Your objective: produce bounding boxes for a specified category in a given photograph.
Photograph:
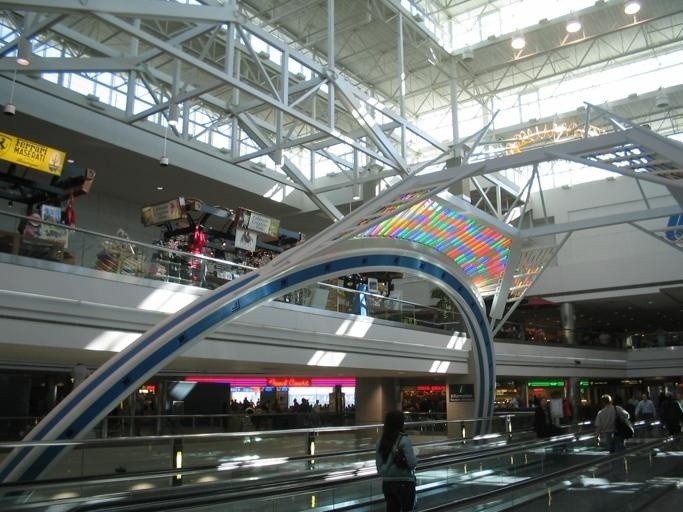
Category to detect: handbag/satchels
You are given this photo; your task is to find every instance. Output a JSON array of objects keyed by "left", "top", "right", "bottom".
[
  {"left": 617, "top": 416, "right": 634, "bottom": 439},
  {"left": 393, "top": 446, "right": 407, "bottom": 468}
]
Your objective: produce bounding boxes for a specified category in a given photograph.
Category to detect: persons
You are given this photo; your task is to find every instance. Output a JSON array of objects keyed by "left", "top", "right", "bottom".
[
  {"left": 230, "top": 397, "right": 355, "bottom": 412},
  {"left": 535, "top": 398, "right": 548, "bottom": 437},
  {"left": 376, "top": 411, "right": 416, "bottom": 512},
  {"left": 352, "top": 283, "right": 367, "bottom": 315},
  {"left": 657, "top": 392, "right": 683, "bottom": 434},
  {"left": 593, "top": 394, "right": 630, "bottom": 448},
  {"left": 635, "top": 393, "right": 656, "bottom": 418}
]
